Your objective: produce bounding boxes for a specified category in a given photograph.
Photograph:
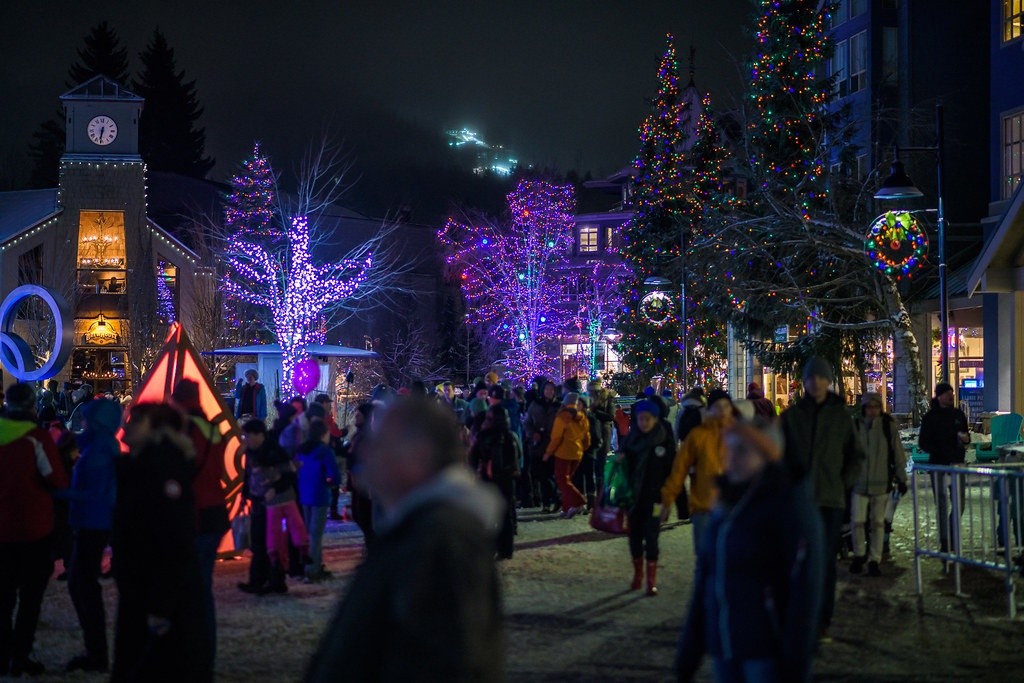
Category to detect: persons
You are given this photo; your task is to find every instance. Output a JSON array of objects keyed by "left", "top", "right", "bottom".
[
  {"left": 234, "top": 369, "right": 267, "bottom": 427},
  {"left": 918, "top": 383, "right": 971, "bottom": 566},
  {"left": 287, "top": 390, "right": 508, "bottom": 683},
  {"left": 240, "top": 380, "right": 428, "bottom": 602},
  {"left": 674, "top": 418, "right": 823, "bottom": 683},
  {"left": 0, "top": 377, "right": 229, "bottom": 683},
  {"left": 109, "top": 277, "right": 122, "bottom": 292},
  {"left": 431, "top": 370, "right": 616, "bottom": 559},
  {"left": 617, "top": 359, "right": 908, "bottom": 595}
]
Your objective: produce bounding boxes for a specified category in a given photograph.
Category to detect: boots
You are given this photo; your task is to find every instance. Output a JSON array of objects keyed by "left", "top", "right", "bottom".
[
  {"left": 630, "top": 557, "right": 644, "bottom": 588},
  {"left": 647, "top": 559, "right": 659, "bottom": 597}
]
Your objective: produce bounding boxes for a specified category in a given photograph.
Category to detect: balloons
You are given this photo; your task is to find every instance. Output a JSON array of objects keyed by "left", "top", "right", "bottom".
[
  {"left": 291, "top": 359, "right": 321, "bottom": 397},
  {"left": 864, "top": 210, "right": 929, "bottom": 282},
  {"left": 637, "top": 292, "right": 677, "bottom": 330}
]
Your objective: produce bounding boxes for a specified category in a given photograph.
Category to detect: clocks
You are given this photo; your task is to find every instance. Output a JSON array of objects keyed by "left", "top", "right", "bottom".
[{"left": 86, "top": 115, "right": 119, "bottom": 147}]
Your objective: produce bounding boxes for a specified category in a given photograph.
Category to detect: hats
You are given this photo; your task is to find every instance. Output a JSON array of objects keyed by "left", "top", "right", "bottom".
[
  {"left": 801, "top": 356, "right": 833, "bottom": 384},
  {"left": 587, "top": 378, "right": 603, "bottom": 392},
  {"left": 635, "top": 400, "right": 659, "bottom": 417},
  {"left": 563, "top": 393, "right": 578, "bottom": 406},
  {"left": 935, "top": 383, "right": 952, "bottom": 396},
  {"left": 728, "top": 416, "right": 785, "bottom": 464},
  {"left": 485, "top": 371, "right": 498, "bottom": 384},
  {"left": 488, "top": 387, "right": 503, "bottom": 399}
]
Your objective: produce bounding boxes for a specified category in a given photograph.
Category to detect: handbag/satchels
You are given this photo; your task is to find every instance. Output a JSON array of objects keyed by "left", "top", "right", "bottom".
[{"left": 588, "top": 481, "right": 631, "bottom": 534}]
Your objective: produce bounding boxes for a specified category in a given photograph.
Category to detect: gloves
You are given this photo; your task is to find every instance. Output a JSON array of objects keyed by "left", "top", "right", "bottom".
[{"left": 897, "top": 481, "right": 908, "bottom": 496}]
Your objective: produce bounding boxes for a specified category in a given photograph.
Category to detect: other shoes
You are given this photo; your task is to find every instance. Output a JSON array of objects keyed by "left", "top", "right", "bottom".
[
  {"left": 9, "top": 657, "right": 46, "bottom": 677},
  {"left": 541, "top": 506, "right": 552, "bottom": 514},
  {"left": 67, "top": 656, "right": 108, "bottom": 672},
  {"left": 496, "top": 553, "right": 512, "bottom": 561},
  {"left": 850, "top": 559, "right": 879, "bottom": 576},
  {"left": 237, "top": 580, "right": 288, "bottom": 596},
  {"left": 881, "top": 553, "right": 896, "bottom": 565},
  {"left": 560, "top": 501, "right": 587, "bottom": 518}
]
